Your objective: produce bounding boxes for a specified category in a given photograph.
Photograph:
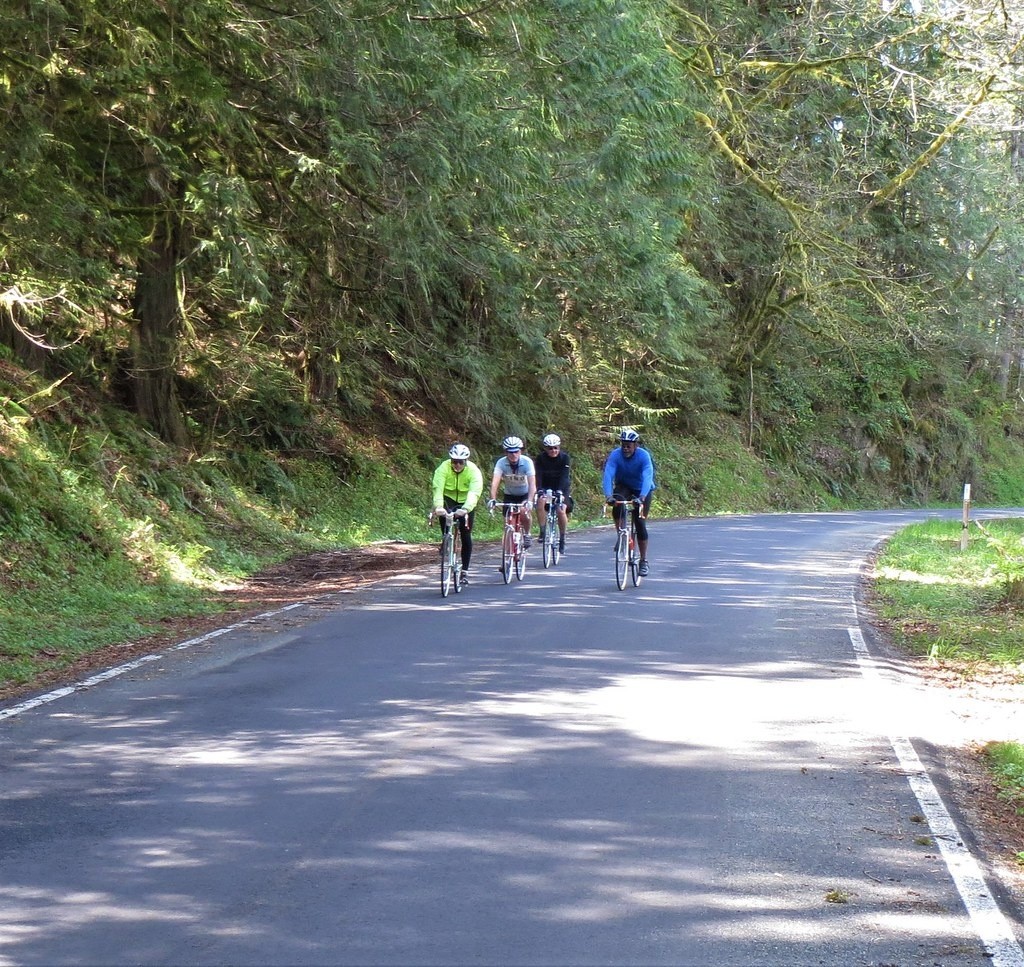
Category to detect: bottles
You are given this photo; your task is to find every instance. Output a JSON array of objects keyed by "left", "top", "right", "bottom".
[{"left": 629, "top": 538, "right": 634, "bottom": 551}]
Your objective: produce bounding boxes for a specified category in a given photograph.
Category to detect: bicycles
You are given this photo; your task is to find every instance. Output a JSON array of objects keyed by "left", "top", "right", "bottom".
[
  {"left": 601, "top": 499, "right": 647, "bottom": 592},
  {"left": 533, "top": 489, "right": 566, "bottom": 567},
  {"left": 486, "top": 499, "right": 534, "bottom": 584},
  {"left": 428, "top": 508, "right": 471, "bottom": 596}
]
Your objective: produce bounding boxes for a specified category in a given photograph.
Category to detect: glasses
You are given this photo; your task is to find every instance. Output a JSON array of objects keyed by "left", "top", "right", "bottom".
[
  {"left": 546, "top": 446, "right": 557, "bottom": 450},
  {"left": 506, "top": 451, "right": 518, "bottom": 453},
  {"left": 453, "top": 460, "right": 464, "bottom": 464},
  {"left": 622, "top": 444, "right": 635, "bottom": 448}
]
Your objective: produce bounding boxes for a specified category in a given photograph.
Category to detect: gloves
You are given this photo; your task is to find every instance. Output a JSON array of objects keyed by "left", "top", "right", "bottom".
[
  {"left": 635, "top": 496, "right": 646, "bottom": 504},
  {"left": 606, "top": 497, "right": 616, "bottom": 506}
]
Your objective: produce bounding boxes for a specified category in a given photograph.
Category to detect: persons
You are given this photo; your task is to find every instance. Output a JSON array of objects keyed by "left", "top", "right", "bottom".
[
  {"left": 432, "top": 444, "right": 483, "bottom": 584},
  {"left": 534, "top": 434, "right": 570, "bottom": 554},
  {"left": 602, "top": 429, "right": 657, "bottom": 576},
  {"left": 490, "top": 436, "right": 536, "bottom": 573}
]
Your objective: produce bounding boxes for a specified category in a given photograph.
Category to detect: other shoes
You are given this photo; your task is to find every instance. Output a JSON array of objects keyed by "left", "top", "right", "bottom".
[
  {"left": 639, "top": 559, "right": 650, "bottom": 576},
  {"left": 524, "top": 534, "right": 532, "bottom": 548},
  {"left": 538, "top": 531, "right": 546, "bottom": 542},
  {"left": 559, "top": 535, "right": 566, "bottom": 554},
  {"left": 440, "top": 541, "right": 448, "bottom": 556},
  {"left": 499, "top": 563, "right": 508, "bottom": 573},
  {"left": 614, "top": 535, "right": 626, "bottom": 551},
  {"left": 460, "top": 571, "right": 468, "bottom": 584}
]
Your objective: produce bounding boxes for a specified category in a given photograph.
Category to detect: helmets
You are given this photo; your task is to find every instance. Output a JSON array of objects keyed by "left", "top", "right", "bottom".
[
  {"left": 621, "top": 430, "right": 639, "bottom": 441},
  {"left": 449, "top": 445, "right": 470, "bottom": 460},
  {"left": 503, "top": 437, "right": 523, "bottom": 451},
  {"left": 543, "top": 434, "right": 561, "bottom": 446}
]
